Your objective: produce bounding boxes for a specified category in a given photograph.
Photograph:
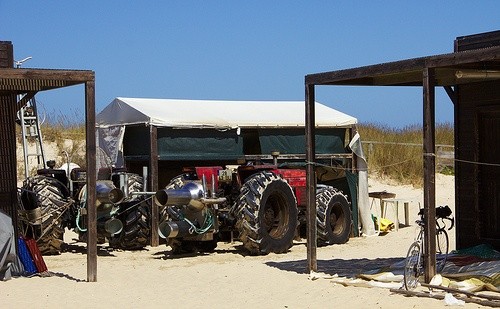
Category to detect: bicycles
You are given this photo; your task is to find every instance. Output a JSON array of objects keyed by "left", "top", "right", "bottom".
[{"left": 399, "top": 205, "right": 455, "bottom": 292}]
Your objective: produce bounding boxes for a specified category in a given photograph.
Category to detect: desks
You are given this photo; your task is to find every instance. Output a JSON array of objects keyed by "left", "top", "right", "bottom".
[
  {"left": 368, "top": 191, "right": 397, "bottom": 221},
  {"left": 382, "top": 198, "right": 421, "bottom": 231}
]
